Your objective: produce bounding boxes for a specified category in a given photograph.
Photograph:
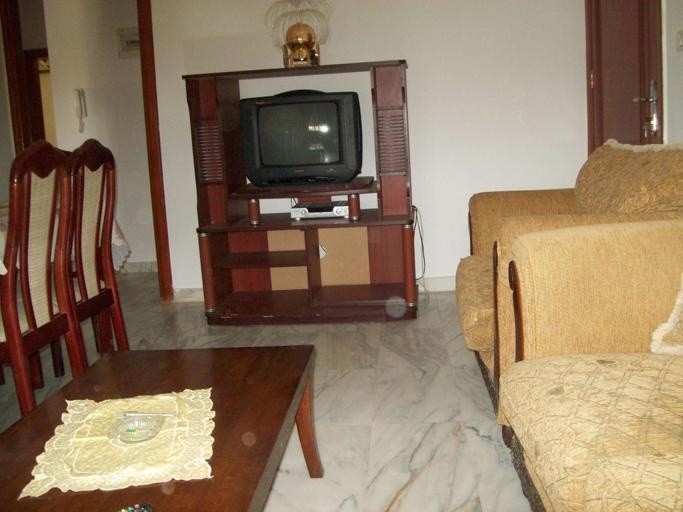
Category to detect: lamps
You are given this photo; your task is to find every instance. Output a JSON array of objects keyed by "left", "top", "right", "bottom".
[{"left": 261, "top": 0, "right": 334, "bottom": 70}]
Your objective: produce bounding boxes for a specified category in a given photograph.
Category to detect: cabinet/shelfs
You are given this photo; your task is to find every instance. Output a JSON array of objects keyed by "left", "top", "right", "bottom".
[{"left": 172, "top": 58, "right": 419, "bottom": 329}]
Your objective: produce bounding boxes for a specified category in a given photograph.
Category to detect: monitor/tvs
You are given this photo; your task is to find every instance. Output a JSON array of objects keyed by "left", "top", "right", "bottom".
[{"left": 238, "top": 89, "right": 363, "bottom": 188}]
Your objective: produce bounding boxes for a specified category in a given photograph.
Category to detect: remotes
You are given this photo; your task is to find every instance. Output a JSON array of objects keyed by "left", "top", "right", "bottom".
[{"left": 119, "top": 502, "right": 155, "bottom": 512}]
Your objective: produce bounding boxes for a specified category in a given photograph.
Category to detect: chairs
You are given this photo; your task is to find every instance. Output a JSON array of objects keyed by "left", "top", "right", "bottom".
[{"left": 1, "top": 137, "right": 131, "bottom": 420}]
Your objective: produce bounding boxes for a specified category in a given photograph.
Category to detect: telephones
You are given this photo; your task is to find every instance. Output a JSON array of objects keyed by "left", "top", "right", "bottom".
[{"left": 72, "top": 88, "right": 87, "bottom": 119}]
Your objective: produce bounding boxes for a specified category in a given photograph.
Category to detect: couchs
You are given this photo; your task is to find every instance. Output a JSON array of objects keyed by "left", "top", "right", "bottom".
[
  {"left": 499, "top": 217, "right": 683, "bottom": 512},
  {"left": 453, "top": 140, "right": 683, "bottom": 422}
]
